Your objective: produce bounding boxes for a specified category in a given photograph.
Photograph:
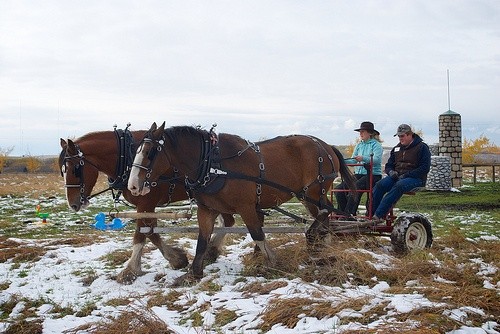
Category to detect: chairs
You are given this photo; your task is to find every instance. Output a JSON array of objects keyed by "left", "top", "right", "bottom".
[{"left": 384, "top": 185, "right": 423, "bottom": 220}]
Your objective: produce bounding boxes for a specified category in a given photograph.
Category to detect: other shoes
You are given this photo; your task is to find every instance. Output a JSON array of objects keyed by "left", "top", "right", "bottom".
[
  {"left": 338, "top": 215, "right": 347, "bottom": 221},
  {"left": 348, "top": 215, "right": 354, "bottom": 219},
  {"left": 372, "top": 215, "right": 384, "bottom": 225}
]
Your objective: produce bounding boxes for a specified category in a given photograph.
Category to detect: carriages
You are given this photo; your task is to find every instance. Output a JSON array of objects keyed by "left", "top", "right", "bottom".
[{"left": 58, "top": 122, "right": 434, "bottom": 284}]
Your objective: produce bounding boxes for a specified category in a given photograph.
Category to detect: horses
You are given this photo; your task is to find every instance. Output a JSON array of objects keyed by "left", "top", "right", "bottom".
[
  {"left": 58, "top": 129, "right": 265, "bottom": 285},
  {"left": 127, "top": 121, "right": 359, "bottom": 279}
]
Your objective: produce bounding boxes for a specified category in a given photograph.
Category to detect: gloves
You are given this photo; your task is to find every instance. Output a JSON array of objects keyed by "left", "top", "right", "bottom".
[
  {"left": 398, "top": 174, "right": 407, "bottom": 180},
  {"left": 388, "top": 170, "right": 399, "bottom": 180}
]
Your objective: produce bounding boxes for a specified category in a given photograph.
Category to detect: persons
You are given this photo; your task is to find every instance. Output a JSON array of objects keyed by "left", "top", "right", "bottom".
[
  {"left": 334, "top": 121, "right": 384, "bottom": 220},
  {"left": 365, "top": 124, "right": 431, "bottom": 222}
]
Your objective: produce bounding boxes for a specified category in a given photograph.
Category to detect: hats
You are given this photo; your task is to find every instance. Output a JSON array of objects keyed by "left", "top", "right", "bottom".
[
  {"left": 394, "top": 124, "right": 411, "bottom": 137},
  {"left": 354, "top": 122, "right": 380, "bottom": 135}
]
